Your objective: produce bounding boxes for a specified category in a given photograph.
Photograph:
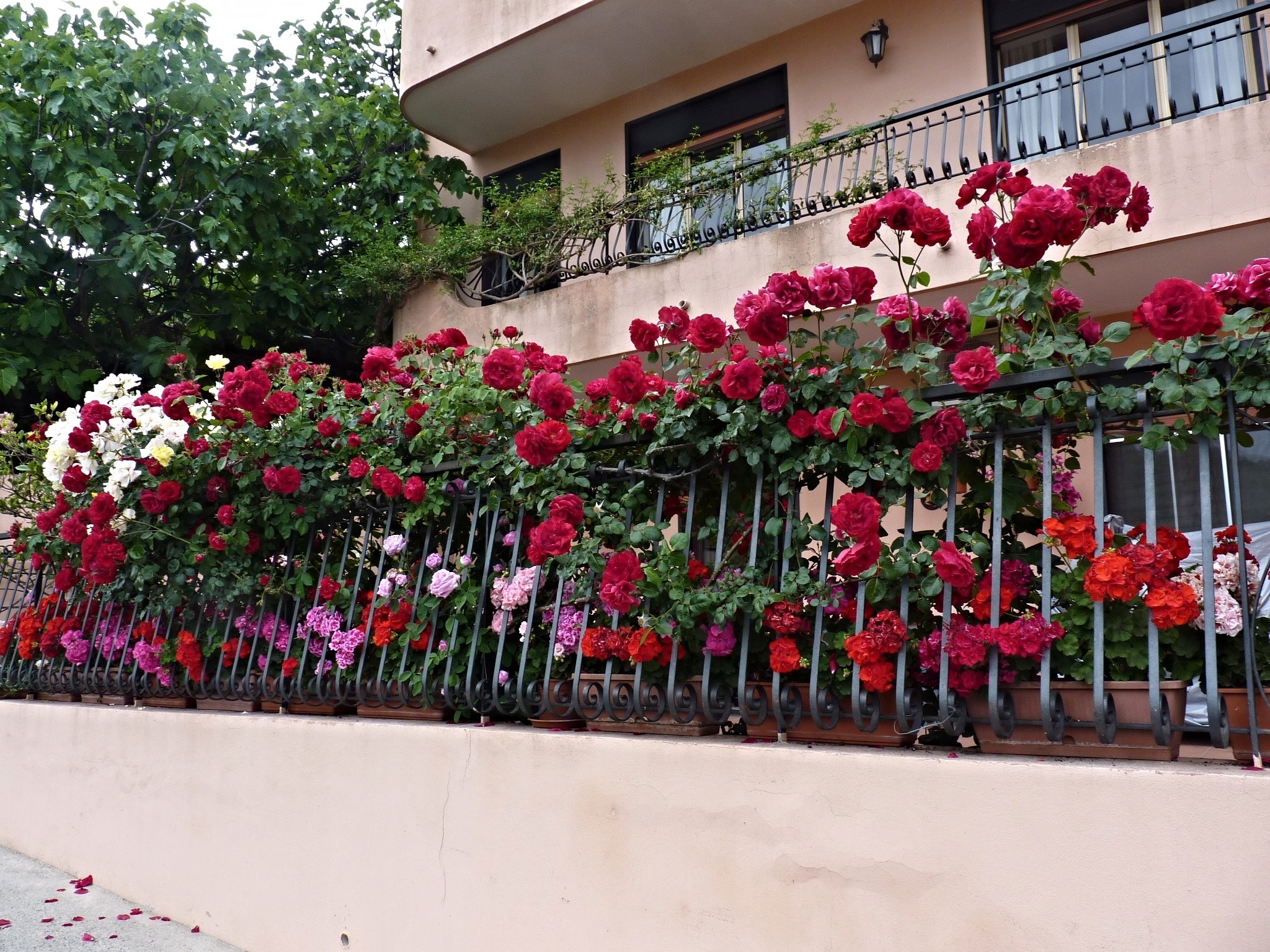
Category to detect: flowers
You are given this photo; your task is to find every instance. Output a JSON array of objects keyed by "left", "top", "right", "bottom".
[{"left": 0, "top": 161, "right": 1270, "bottom": 722}]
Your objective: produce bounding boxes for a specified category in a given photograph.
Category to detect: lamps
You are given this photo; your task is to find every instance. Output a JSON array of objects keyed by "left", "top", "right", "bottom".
[{"left": 860, "top": 19, "right": 889, "bottom": 69}]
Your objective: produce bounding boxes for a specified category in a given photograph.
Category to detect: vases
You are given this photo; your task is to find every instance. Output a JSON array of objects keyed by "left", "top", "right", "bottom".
[
  {"left": 527, "top": 679, "right": 586, "bottom": 730},
  {"left": 1217, "top": 689, "right": 1270, "bottom": 765},
  {"left": 34, "top": 666, "right": 77, "bottom": 702},
  {"left": 354, "top": 674, "right": 462, "bottom": 722},
  {"left": 193, "top": 669, "right": 258, "bottom": 712},
  {"left": 966, "top": 681, "right": 1187, "bottom": 761},
  {"left": 572, "top": 673, "right": 721, "bottom": 736},
  {"left": 76, "top": 667, "right": 134, "bottom": 705},
  {"left": 745, "top": 682, "right": 915, "bottom": 746},
  {"left": 255, "top": 672, "right": 337, "bottom": 716},
  {"left": 134, "top": 673, "right": 191, "bottom": 708}
]
